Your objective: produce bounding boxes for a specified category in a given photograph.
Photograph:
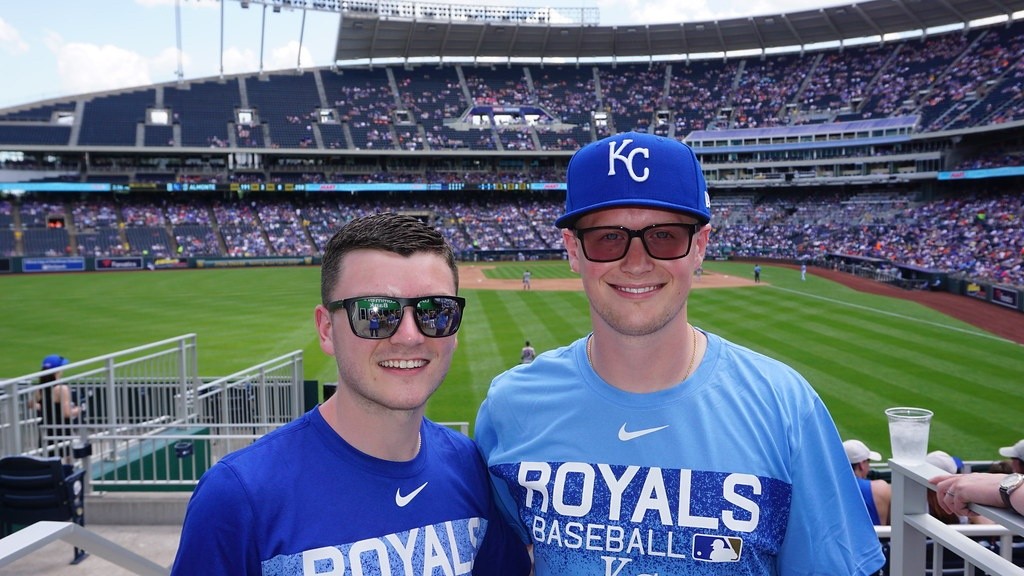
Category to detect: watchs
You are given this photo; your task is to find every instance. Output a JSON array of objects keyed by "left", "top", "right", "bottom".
[{"left": 999, "top": 472, "right": 1024, "bottom": 513}]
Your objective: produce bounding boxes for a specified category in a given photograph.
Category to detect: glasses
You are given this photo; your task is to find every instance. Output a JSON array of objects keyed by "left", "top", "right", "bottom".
[
  {"left": 574, "top": 224, "right": 702, "bottom": 262},
  {"left": 325, "top": 295, "right": 466, "bottom": 339}
]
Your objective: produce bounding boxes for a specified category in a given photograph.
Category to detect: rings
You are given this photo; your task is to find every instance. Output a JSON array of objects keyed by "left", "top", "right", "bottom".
[{"left": 947, "top": 490, "right": 954, "bottom": 496}]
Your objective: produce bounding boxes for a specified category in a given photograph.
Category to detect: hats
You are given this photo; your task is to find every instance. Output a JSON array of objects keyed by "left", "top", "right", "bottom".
[
  {"left": 999, "top": 439, "right": 1024, "bottom": 460},
  {"left": 555, "top": 133, "right": 709, "bottom": 226},
  {"left": 843, "top": 439, "right": 881, "bottom": 463},
  {"left": 43, "top": 355, "right": 68, "bottom": 367},
  {"left": 927, "top": 451, "right": 964, "bottom": 474}
]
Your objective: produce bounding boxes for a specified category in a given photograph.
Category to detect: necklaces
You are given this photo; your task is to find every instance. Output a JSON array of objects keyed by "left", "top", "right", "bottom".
[{"left": 588, "top": 323, "right": 697, "bottom": 383}]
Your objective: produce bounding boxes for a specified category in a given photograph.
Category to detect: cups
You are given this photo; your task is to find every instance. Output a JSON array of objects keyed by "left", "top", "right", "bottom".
[{"left": 885, "top": 407, "right": 933, "bottom": 466}]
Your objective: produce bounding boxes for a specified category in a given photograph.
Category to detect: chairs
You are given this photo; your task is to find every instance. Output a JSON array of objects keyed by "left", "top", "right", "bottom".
[{"left": 0, "top": 456, "right": 90, "bottom": 565}]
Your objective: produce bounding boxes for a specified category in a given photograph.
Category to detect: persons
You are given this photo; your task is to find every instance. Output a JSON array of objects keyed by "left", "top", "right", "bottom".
[
  {"left": 754, "top": 264, "right": 761, "bottom": 282},
  {"left": 168, "top": 29, "right": 1024, "bottom": 151},
  {"left": 842, "top": 439, "right": 891, "bottom": 525},
  {"left": 697, "top": 267, "right": 703, "bottom": 280},
  {"left": 0, "top": 155, "right": 1024, "bottom": 289},
  {"left": 929, "top": 472, "right": 1024, "bottom": 517},
  {"left": 170, "top": 210, "right": 532, "bottom": 576},
  {"left": 473, "top": 129, "right": 886, "bottom": 576},
  {"left": 27, "top": 354, "right": 82, "bottom": 457},
  {"left": 926, "top": 450, "right": 964, "bottom": 570},
  {"left": 998, "top": 440, "right": 1024, "bottom": 474},
  {"left": 422, "top": 309, "right": 459, "bottom": 335},
  {"left": 369, "top": 308, "right": 398, "bottom": 337},
  {"left": 801, "top": 262, "right": 806, "bottom": 280},
  {"left": 521, "top": 341, "right": 535, "bottom": 363},
  {"left": 523, "top": 269, "right": 530, "bottom": 290}
]
